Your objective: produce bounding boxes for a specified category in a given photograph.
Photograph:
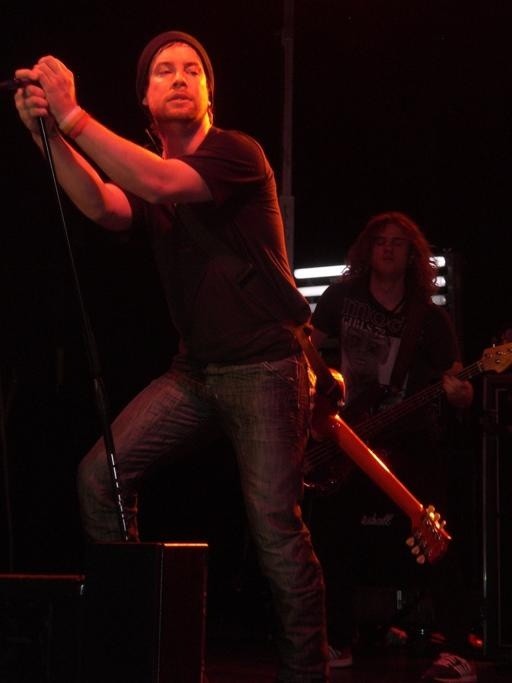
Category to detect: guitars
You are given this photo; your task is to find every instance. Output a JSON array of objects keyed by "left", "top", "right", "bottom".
[
  {"left": 306, "top": 333, "right": 453, "bottom": 565},
  {"left": 302, "top": 339, "right": 512, "bottom": 506}
]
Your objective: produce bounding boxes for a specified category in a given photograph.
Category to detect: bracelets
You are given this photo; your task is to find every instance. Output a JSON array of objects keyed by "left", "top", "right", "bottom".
[{"left": 60, "top": 105, "right": 92, "bottom": 141}]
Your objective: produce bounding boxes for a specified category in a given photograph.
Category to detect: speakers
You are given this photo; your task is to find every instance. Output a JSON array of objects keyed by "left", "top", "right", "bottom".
[
  {"left": 0, "top": 573, "right": 86, "bottom": 682},
  {"left": 85, "top": 539, "right": 210, "bottom": 682}
]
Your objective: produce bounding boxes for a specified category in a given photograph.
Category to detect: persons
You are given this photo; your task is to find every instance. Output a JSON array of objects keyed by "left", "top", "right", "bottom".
[
  {"left": 302, "top": 212, "right": 488, "bottom": 682},
  {"left": 11, "top": 30, "right": 330, "bottom": 680}
]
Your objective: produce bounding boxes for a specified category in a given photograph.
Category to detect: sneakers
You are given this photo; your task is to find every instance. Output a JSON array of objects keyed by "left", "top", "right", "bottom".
[
  {"left": 327, "top": 645, "right": 354, "bottom": 668},
  {"left": 422, "top": 652, "right": 478, "bottom": 683}
]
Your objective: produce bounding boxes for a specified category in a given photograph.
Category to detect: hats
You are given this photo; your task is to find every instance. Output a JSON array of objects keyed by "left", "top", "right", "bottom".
[{"left": 135, "top": 30, "right": 216, "bottom": 107}]
